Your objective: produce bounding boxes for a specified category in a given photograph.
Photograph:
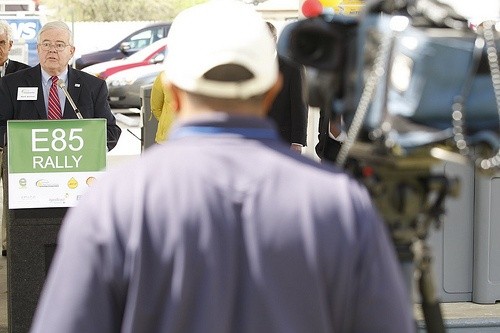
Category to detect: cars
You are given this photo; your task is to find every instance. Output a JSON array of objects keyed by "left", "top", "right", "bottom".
[
  {"left": 70, "top": 23, "right": 172, "bottom": 75},
  {"left": 77, "top": 35, "right": 166, "bottom": 111}
]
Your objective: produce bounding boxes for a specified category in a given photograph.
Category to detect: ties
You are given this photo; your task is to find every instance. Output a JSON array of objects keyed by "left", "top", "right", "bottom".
[{"left": 47, "top": 75, "right": 63, "bottom": 121}]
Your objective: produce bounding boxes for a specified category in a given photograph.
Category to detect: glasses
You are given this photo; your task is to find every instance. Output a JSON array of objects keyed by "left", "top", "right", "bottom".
[{"left": 38, "top": 42, "right": 72, "bottom": 51}]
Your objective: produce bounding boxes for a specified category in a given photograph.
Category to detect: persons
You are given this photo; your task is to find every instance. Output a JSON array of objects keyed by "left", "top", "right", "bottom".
[
  {"left": 148, "top": 71, "right": 173, "bottom": 144},
  {"left": 31, "top": 0, "right": 418, "bottom": 333},
  {"left": 315, "top": 107, "right": 355, "bottom": 166},
  {"left": 0, "top": 21, "right": 122, "bottom": 152},
  {"left": 266, "top": 22, "right": 308, "bottom": 156},
  {"left": 0, "top": 19, "right": 31, "bottom": 256}
]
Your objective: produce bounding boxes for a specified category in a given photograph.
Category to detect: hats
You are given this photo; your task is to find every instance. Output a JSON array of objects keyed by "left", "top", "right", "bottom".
[{"left": 164, "top": 0, "right": 279, "bottom": 100}]
